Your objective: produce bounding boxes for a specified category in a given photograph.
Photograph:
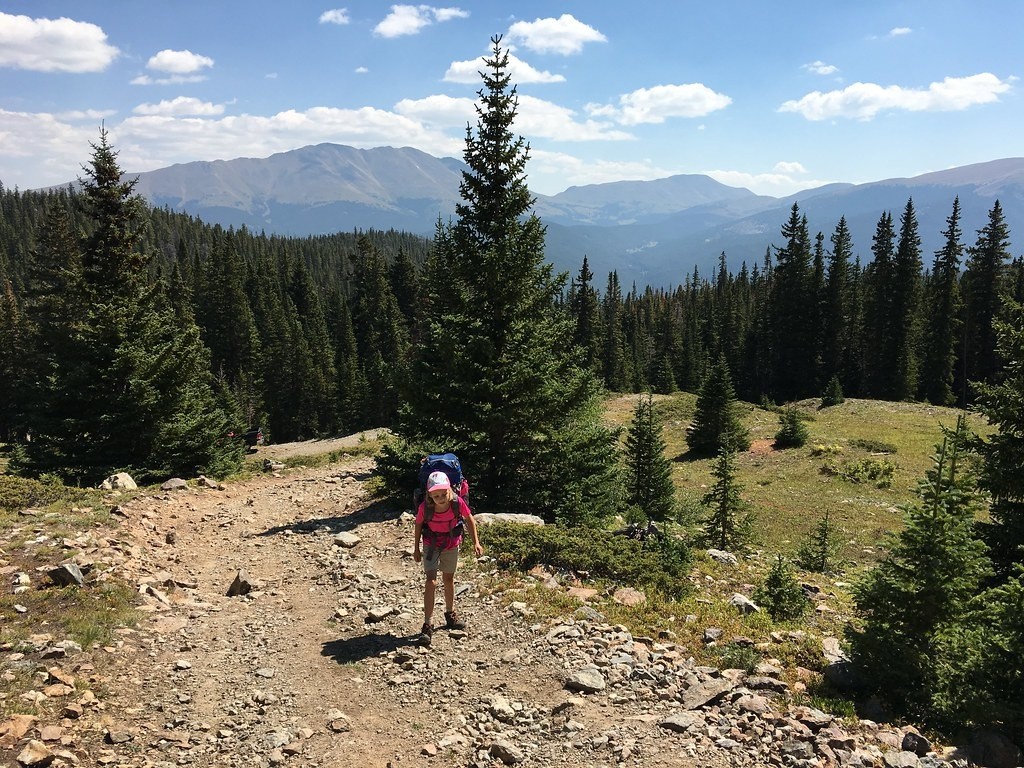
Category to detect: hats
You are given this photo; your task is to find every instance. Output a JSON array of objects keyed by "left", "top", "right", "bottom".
[{"left": 427, "top": 471, "right": 450, "bottom": 492}]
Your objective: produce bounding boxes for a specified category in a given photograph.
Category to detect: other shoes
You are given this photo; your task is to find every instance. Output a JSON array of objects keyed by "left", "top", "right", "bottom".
[
  {"left": 419, "top": 623, "right": 432, "bottom": 644},
  {"left": 444, "top": 613, "right": 465, "bottom": 629}
]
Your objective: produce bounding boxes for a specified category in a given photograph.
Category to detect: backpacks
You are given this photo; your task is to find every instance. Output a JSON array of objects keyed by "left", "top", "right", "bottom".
[{"left": 419, "top": 453, "right": 469, "bottom": 537}]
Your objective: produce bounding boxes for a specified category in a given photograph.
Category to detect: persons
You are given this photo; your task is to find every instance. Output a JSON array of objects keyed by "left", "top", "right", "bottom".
[{"left": 413, "top": 472, "right": 483, "bottom": 644}]
